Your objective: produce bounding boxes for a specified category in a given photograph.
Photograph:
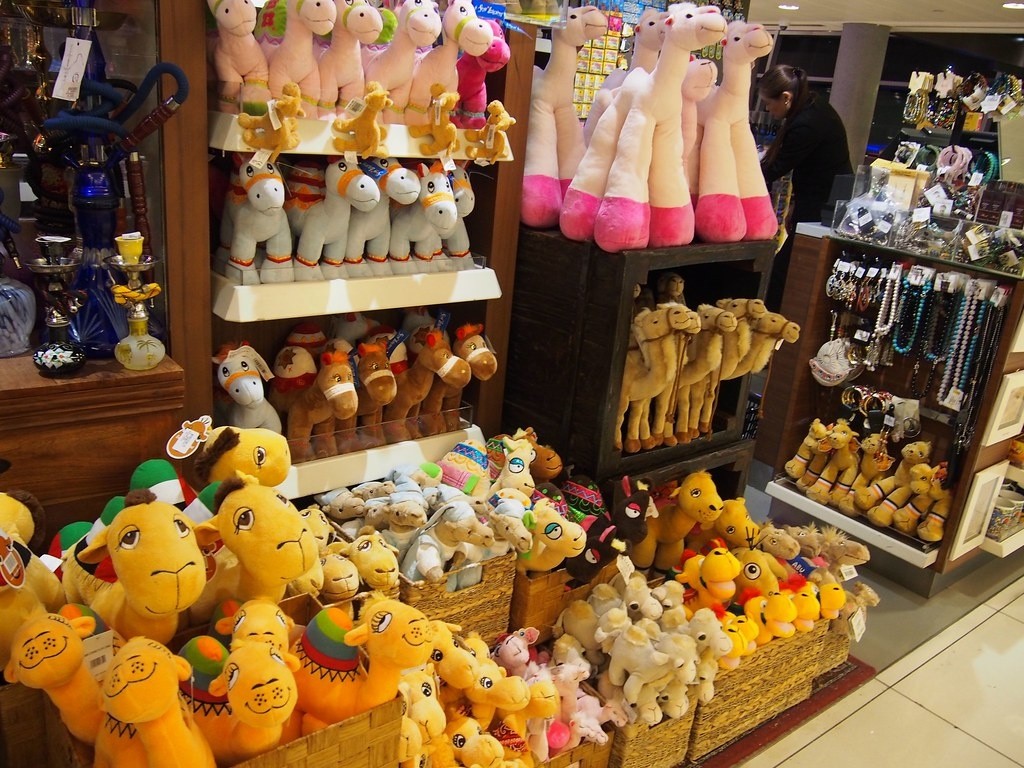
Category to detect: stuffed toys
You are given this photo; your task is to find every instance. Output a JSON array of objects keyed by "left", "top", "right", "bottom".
[
  {"left": 211, "top": 306, "right": 498, "bottom": 465},
  {"left": 208, "top": 0, "right": 517, "bottom": 285},
  {"left": 0, "top": 425, "right": 881, "bottom": 768},
  {"left": 520, "top": 0, "right": 801, "bottom": 458},
  {"left": 785, "top": 419, "right": 957, "bottom": 541}
]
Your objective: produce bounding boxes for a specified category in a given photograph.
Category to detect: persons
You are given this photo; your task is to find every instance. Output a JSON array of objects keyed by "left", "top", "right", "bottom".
[{"left": 758, "top": 64, "right": 854, "bottom": 369}]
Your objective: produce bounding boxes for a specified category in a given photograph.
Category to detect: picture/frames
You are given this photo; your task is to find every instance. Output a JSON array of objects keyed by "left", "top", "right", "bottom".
[
  {"left": 1010, "top": 309, "right": 1024, "bottom": 352},
  {"left": 949, "top": 459, "right": 1011, "bottom": 562},
  {"left": 980, "top": 370, "right": 1024, "bottom": 447}
]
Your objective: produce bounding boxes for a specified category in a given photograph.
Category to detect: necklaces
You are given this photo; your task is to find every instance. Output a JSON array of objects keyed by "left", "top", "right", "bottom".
[{"left": 848, "top": 267, "right": 1004, "bottom": 454}]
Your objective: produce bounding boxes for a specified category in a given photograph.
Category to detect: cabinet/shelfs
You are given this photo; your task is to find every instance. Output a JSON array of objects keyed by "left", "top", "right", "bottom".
[
  {"left": 159, "top": 0, "right": 539, "bottom": 501},
  {"left": 0, "top": 1, "right": 184, "bottom": 555}
]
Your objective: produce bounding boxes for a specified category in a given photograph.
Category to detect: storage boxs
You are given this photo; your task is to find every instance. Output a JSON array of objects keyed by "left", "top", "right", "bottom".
[
  {"left": 573, "top": 0, "right": 667, "bottom": 117},
  {"left": 829, "top": 165, "right": 1024, "bottom": 278},
  {"left": 979, "top": 465, "right": 1024, "bottom": 559}
]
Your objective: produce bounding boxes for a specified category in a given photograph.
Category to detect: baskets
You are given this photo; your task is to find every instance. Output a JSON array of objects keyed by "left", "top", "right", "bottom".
[{"left": 0, "top": 533, "right": 853, "bottom": 768}]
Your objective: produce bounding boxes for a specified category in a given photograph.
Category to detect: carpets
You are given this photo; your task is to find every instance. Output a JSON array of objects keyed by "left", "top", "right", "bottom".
[{"left": 697, "top": 654, "right": 877, "bottom": 768}]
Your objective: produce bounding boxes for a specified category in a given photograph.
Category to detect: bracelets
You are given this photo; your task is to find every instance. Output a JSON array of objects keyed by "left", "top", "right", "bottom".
[{"left": 842, "top": 385, "right": 921, "bottom": 438}]
